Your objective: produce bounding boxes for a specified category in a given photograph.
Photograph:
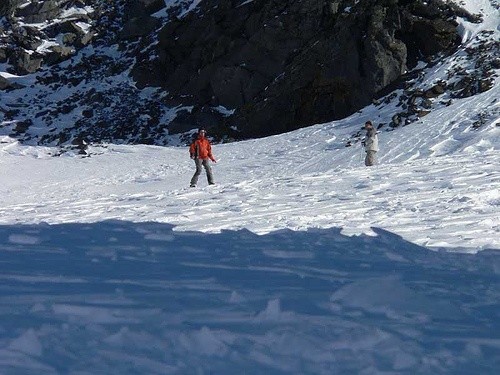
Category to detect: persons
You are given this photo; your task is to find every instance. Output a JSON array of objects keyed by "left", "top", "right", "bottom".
[
  {"left": 189, "top": 128, "right": 219, "bottom": 189},
  {"left": 362, "top": 120, "right": 380, "bottom": 167}
]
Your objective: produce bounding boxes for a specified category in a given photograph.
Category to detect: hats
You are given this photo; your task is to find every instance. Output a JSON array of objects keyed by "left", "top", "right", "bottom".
[
  {"left": 199, "top": 127, "right": 207, "bottom": 132},
  {"left": 365, "top": 121, "right": 373, "bottom": 126}
]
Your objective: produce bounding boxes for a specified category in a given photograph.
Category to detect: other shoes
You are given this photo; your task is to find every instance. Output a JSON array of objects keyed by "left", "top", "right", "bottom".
[{"left": 190, "top": 185, "right": 196, "bottom": 187}]
